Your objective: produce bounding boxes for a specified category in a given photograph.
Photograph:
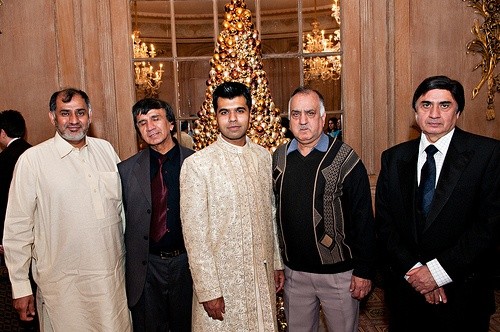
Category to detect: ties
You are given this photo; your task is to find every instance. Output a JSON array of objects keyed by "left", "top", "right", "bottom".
[
  {"left": 150, "top": 155, "right": 168, "bottom": 244},
  {"left": 419, "top": 144, "right": 438, "bottom": 214}
]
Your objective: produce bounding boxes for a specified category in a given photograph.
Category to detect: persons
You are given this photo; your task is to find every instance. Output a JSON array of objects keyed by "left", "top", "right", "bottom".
[
  {"left": 3, "top": 87, "right": 134, "bottom": 332},
  {"left": 175, "top": 114, "right": 200, "bottom": 149},
  {"left": 271, "top": 86, "right": 374, "bottom": 332},
  {"left": 0, "top": 109, "right": 34, "bottom": 332},
  {"left": 117, "top": 98, "right": 197, "bottom": 332},
  {"left": 179, "top": 81, "right": 285, "bottom": 332},
  {"left": 326, "top": 117, "right": 343, "bottom": 137},
  {"left": 373, "top": 76, "right": 500, "bottom": 332}
]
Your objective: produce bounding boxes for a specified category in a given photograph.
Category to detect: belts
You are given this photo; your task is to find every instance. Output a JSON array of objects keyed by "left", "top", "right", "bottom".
[{"left": 149, "top": 250, "right": 185, "bottom": 259}]
